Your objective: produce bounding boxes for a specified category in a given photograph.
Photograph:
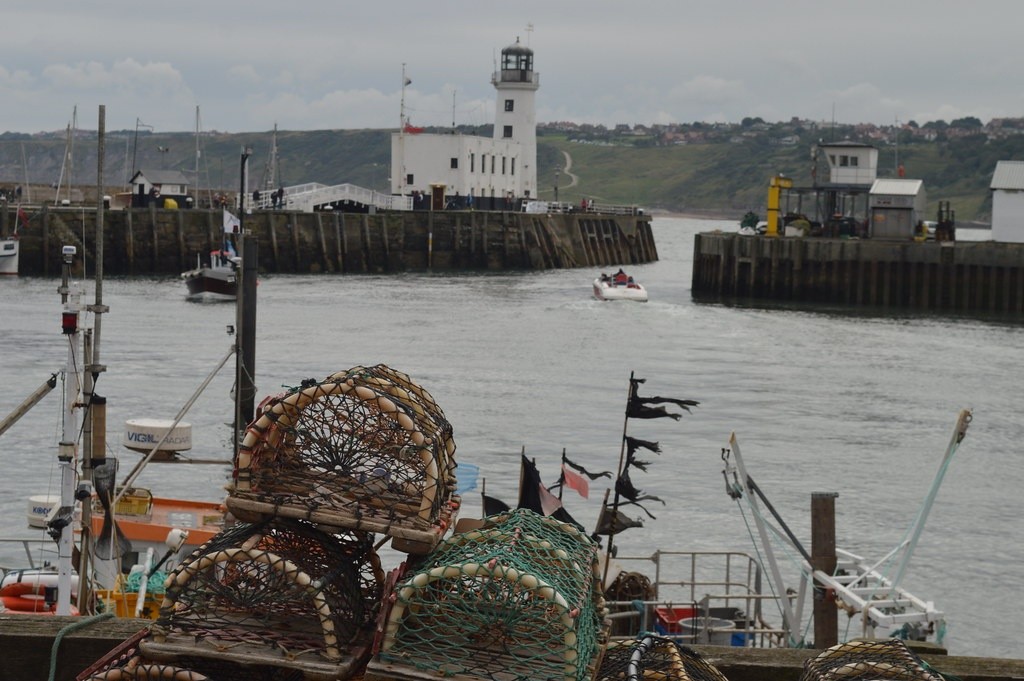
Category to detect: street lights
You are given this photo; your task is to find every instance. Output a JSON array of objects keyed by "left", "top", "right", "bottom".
[{"left": 158, "top": 146, "right": 169, "bottom": 171}]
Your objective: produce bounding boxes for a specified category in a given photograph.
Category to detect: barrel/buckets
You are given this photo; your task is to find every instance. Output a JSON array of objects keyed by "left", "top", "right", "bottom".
[{"left": 678, "top": 616, "right": 736, "bottom": 646}]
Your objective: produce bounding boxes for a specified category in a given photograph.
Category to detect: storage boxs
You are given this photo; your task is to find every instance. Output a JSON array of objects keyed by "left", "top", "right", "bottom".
[{"left": 655, "top": 606, "right": 755, "bottom": 647}]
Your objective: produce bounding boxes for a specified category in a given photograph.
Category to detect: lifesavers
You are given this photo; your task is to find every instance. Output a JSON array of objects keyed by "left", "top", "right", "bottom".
[
  {"left": 1, "top": 604, "right": 80, "bottom": 617},
  {"left": 1, "top": 581, "right": 60, "bottom": 611}
]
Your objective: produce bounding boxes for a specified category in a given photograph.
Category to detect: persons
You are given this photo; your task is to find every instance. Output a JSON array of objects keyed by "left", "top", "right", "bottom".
[
  {"left": 252, "top": 190, "right": 259, "bottom": 209},
  {"left": 581, "top": 198, "right": 588, "bottom": 208},
  {"left": 410, "top": 190, "right": 427, "bottom": 209},
  {"left": 465, "top": 193, "right": 474, "bottom": 210},
  {"left": 613, "top": 267, "right": 625, "bottom": 276},
  {"left": 212, "top": 190, "right": 229, "bottom": 209},
  {"left": 269, "top": 186, "right": 284, "bottom": 212},
  {"left": 453, "top": 191, "right": 458, "bottom": 210},
  {"left": 504, "top": 194, "right": 515, "bottom": 210}
]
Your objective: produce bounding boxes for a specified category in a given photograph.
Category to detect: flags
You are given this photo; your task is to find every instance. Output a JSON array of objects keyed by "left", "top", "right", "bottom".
[{"left": 223, "top": 210, "right": 241, "bottom": 234}]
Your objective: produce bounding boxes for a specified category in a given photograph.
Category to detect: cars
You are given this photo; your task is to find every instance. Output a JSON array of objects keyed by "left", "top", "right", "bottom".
[{"left": 924, "top": 220, "right": 938, "bottom": 239}]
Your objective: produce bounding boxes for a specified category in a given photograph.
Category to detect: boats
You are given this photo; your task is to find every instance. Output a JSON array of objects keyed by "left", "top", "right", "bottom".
[
  {"left": 593, "top": 277, "right": 647, "bottom": 302},
  {"left": 0, "top": 102, "right": 1024, "bottom": 681},
  {"left": 0, "top": 202, "right": 34, "bottom": 278},
  {"left": 181, "top": 206, "right": 259, "bottom": 303}
]
